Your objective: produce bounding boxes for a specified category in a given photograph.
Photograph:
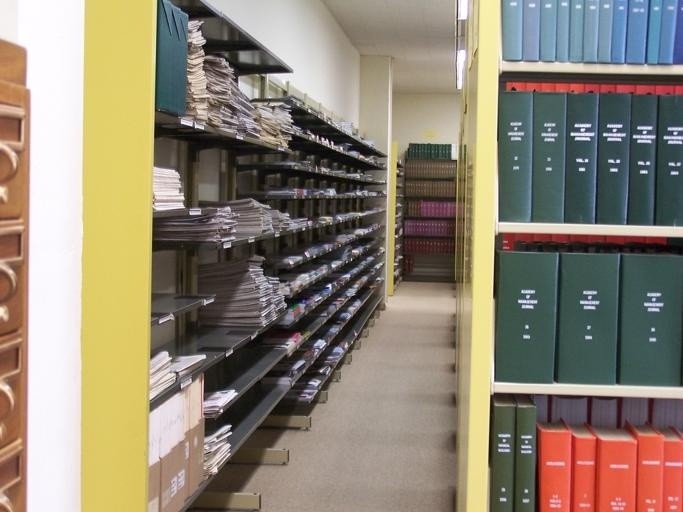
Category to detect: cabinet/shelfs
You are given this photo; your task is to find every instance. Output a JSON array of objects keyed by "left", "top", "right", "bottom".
[
  {"left": 405, "top": 157, "right": 455, "bottom": 282},
  {"left": 455, "top": 1, "right": 683, "bottom": 510},
  {"left": 84, "top": 1, "right": 386, "bottom": 512}
]
